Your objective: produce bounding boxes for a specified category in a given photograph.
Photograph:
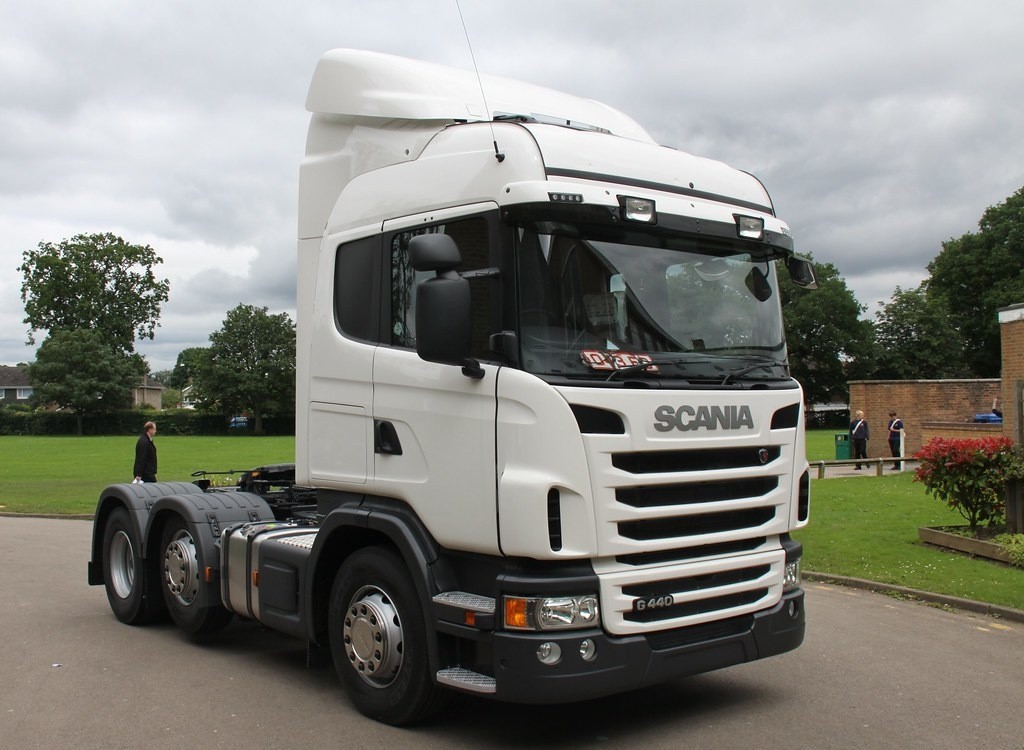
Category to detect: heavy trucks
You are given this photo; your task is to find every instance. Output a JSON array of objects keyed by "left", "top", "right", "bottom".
[{"left": 87, "top": 45, "right": 813, "bottom": 729}]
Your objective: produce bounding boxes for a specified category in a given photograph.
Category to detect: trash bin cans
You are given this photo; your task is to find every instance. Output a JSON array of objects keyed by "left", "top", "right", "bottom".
[{"left": 835, "top": 433, "right": 853, "bottom": 461}]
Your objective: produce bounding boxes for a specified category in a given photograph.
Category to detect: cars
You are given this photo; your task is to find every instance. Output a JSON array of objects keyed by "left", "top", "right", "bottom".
[{"left": 228, "top": 417, "right": 255, "bottom": 430}]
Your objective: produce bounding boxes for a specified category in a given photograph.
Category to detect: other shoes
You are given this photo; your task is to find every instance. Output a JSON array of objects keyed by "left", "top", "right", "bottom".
[
  {"left": 891, "top": 465, "right": 901, "bottom": 470},
  {"left": 854, "top": 466, "right": 861, "bottom": 471},
  {"left": 866, "top": 464, "right": 870, "bottom": 468}
]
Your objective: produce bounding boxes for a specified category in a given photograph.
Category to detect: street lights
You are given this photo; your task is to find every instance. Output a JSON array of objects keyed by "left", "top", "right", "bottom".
[{"left": 180, "top": 363, "right": 186, "bottom": 411}]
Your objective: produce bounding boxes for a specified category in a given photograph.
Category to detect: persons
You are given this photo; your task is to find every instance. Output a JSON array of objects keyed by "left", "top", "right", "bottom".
[
  {"left": 849, "top": 410, "right": 870, "bottom": 470},
  {"left": 133, "top": 421, "right": 158, "bottom": 483},
  {"left": 887, "top": 409, "right": 903, "bottom": 470},
  {"left": 992, "top": 398, "right": 1002, "bottom": 418}
]
